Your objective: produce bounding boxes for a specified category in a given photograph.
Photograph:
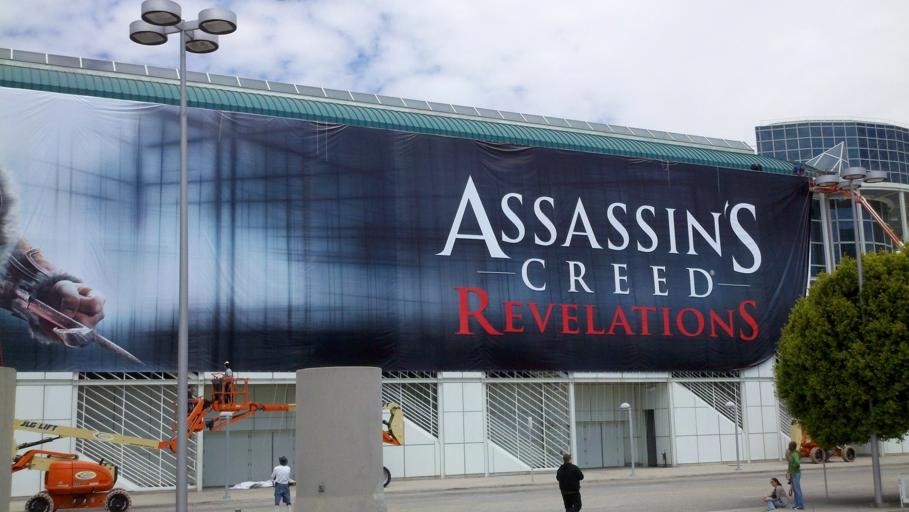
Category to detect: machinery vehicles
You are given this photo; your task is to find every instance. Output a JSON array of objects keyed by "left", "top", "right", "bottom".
[{"left": 11, "top": 372, "right": 404, "bottom": 512}]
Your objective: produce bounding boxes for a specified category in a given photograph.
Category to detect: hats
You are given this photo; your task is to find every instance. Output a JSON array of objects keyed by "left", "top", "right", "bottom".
[
  {"left": 278, "top": 455, "right": 289, "bottom": 465},
  {"left": 223, "top": 361, "right": 231, "bottom": 366}
]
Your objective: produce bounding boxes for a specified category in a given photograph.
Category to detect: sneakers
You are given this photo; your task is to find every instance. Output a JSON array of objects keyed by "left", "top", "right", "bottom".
[{"left": 792, "top": 506, "right": 804, "bottom": 510}]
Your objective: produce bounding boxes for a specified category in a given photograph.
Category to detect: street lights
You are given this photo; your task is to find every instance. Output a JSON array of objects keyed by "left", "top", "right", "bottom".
[
  {"left": 619, "top": 402, "right": 638, "bottom": 477},
  {"left": 128, "top": 0, "right": 237, "bottom": 512},
  {"left": 815, "top": 167, "right": 888, "bottom": 507},
  {"left": 725, "top": 402, "right": 742, "bottom": 471}
]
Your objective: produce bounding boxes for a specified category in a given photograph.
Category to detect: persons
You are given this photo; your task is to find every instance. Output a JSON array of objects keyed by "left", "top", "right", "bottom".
[
  {"left": 223, "top": 360, "right": 233, "bottom": 404},
  {"left": 786, "top": 441, "right": 805, "bottom": 509},
  {"left": 0, "top": 166, "right": 107, "bottom": 349},
  {"left": 269, "top": 456, "right": 293, "bottom": 512},
  {"left": 762, "top": 477, "right": 787, "bottom": 511},
  {"left": 556, "top": 454, "right": 584, "bottom": 511}
]
